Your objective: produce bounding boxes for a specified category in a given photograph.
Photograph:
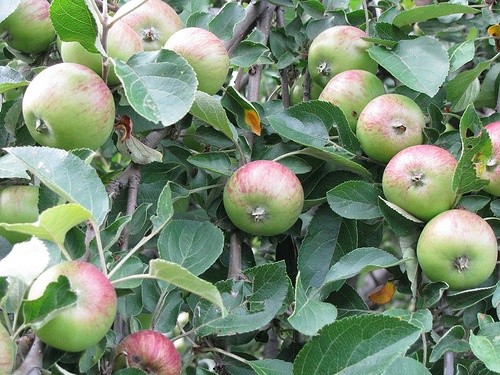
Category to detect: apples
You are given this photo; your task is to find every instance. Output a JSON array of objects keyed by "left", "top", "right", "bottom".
[
  {"left": 0, "top": 0, "right": 229, "bottom": 152},
  {"left": 222, "top": 159, "right": 303, "bottom": 238},
  {"left": 285, "top": 22, "right": 500, "bottom": 290},
  {"left": 0, "top": 184, "right": 184, "bottom": 375}
]
